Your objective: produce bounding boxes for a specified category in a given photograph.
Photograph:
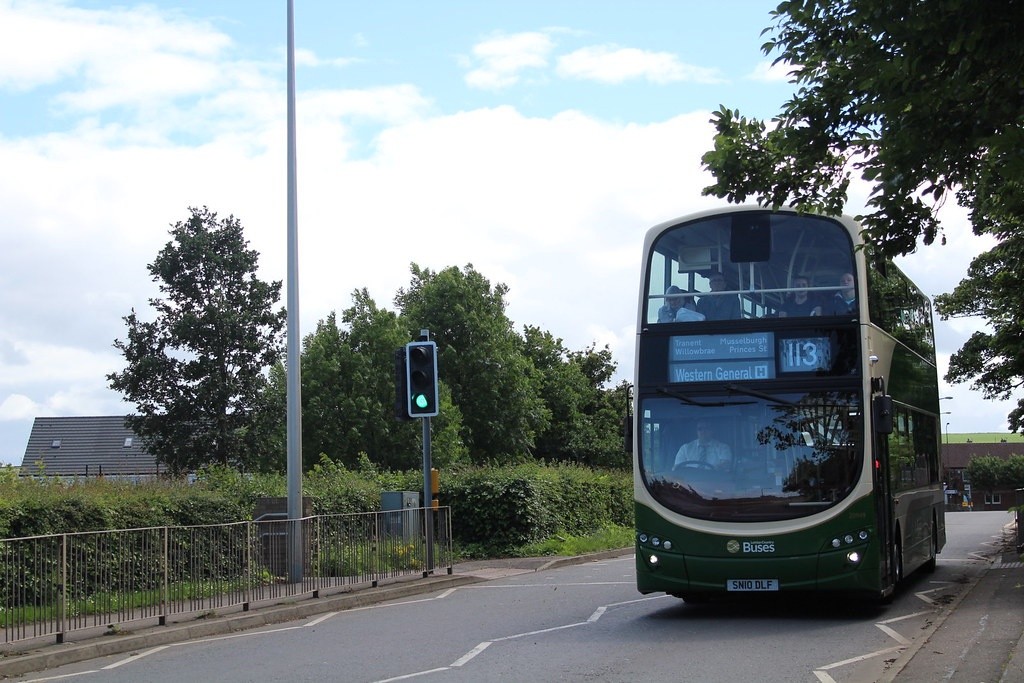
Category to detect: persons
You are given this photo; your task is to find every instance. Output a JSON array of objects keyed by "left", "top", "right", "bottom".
[
  {"left": 696, "top": 273, "right": 740, "bottom": 321},
  {"left": 823, "top": 272, "right": 856, "bottom": 316},
  {"left": 657, "top": 286, "right": 695, "bottom": 324},
  {"left": 779, "top": 277, "right": 823, "bottom": 316},
  {"left": 672, "top": 418, "right": 731, "bottom": 472}
]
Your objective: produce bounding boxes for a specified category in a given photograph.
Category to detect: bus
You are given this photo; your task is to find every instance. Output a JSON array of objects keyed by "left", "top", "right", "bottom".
[{"left": 623, "top": 203, "right": 947, "bottom": 607}]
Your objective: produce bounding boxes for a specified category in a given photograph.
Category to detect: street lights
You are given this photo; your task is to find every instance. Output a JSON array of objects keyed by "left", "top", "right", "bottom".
[{"left": 945, "top": 422, "right": 950, "bottom": 454}]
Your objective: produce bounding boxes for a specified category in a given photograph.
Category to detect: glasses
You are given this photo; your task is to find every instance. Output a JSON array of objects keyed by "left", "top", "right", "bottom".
[{"left": 697, "top": 426, "right": 712, "bottom": 431}]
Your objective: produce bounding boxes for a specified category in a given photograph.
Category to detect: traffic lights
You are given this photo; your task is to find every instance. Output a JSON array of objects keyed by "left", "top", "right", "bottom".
[{"left": 406, "top": 341, "right": 440, "bottom": 418}]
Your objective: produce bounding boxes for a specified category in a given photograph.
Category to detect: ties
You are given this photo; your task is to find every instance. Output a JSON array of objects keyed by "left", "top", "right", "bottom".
[{"left": 697, "top": 445, "right": 709, "bottom": 471}]
